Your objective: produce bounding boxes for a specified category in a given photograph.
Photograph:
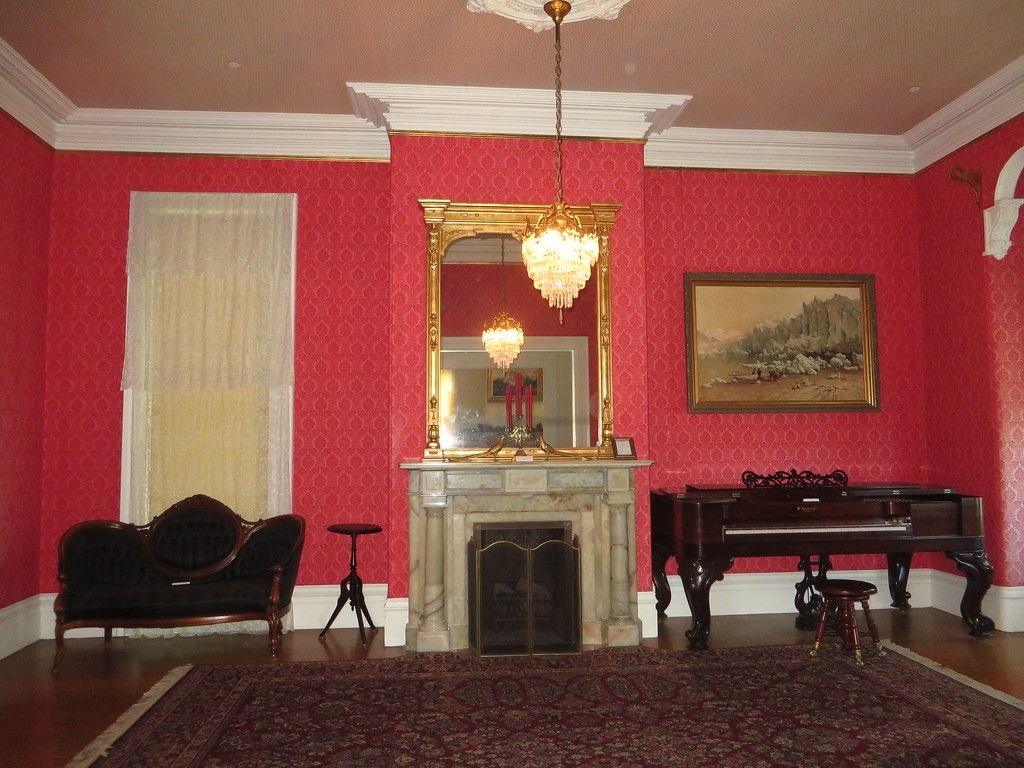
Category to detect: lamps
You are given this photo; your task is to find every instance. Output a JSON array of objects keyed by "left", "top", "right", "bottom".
[
  {"left": 466, "top": 0, "right": 629, "bottom": 325},
  {"left": 482, "top": 239, "right": 525, "bottom": 378}
]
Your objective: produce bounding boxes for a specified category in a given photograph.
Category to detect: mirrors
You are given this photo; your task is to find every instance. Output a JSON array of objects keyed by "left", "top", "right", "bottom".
[{"left": 417, "top": 197, "right": 623, "bottom": 462}]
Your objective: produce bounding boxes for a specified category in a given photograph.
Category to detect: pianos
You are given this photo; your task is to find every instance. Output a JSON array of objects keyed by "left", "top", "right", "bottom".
[{"left": 649, "top": 484, "right": 996, "bottom": 652}]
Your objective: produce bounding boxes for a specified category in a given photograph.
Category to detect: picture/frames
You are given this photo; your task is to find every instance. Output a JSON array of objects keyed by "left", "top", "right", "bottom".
[
  {"left": 485, "top": 367, "right": 543, "bottom": 401},
  {"left": 682, "top": 271, "right": 882, "bottom": 414},
  {"left": 610, "top": 436, "right": 638, "bottom": 460}
]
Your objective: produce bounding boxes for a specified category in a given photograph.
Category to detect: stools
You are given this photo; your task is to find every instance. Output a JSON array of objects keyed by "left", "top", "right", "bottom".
[{"left": 809, "top": 579, "right": 889, "bottom": 666}]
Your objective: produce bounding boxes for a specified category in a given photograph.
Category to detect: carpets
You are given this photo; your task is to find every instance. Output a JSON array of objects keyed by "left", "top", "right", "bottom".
[{"left": 64, "top": 638, "right": 1024, "bottom": 768}]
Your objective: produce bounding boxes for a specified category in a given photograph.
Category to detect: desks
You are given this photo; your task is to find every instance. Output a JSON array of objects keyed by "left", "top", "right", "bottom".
[{"left": 317, "top": 522, "right": 382, "bottom": 648}]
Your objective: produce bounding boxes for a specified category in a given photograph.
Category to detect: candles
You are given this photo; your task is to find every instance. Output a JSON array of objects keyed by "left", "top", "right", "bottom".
[
  {"left": 515, "top": 369, "right": 522, "bottom": 416},
  {"left": 505, "top": 380, "right": 512, "bottom": 428},
  {"left": 524, "top": 383, "right": 532, "bottom": 428}
]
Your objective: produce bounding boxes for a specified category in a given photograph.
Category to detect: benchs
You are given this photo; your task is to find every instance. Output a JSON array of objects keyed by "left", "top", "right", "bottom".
[{"left": 50, "top": 494, "right": 307, "bottom": 677}]
